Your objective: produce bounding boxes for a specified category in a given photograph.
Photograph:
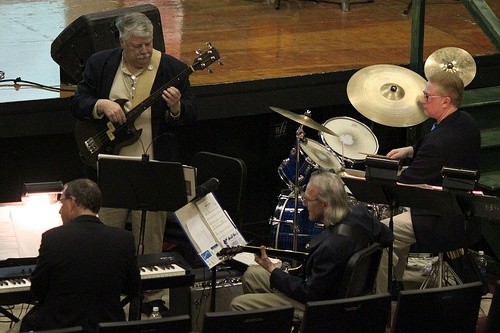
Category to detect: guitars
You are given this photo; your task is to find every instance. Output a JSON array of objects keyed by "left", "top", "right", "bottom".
[
  {"left": 73, "top": 41, "right": 225, "bottom": 167},
  {"left": 216, "top": 245, "right": 310, "bottom": 266}
]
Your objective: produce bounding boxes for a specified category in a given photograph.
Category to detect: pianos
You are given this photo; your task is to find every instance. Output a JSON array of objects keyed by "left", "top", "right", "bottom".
[{"left": 0, "top": 251, "right": 196, "bottom": 329}]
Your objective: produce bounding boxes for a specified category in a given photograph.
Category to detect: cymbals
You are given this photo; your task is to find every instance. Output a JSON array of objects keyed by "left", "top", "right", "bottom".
[
  {"left": 346, "top": 64, "right": 430, "bottom": 128},
  {"left": 269, "top": 106, "right": 340, "bottom": 138},
  {"left": 424, "top": 46, "right": 477, "bottom": 88}
]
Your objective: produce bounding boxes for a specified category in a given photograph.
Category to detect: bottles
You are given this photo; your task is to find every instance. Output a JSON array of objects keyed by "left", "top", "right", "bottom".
[
  {"left": 475, "top": 251, "right": 488, "bottom": 281},
  {"left": 148, "top": 307, "right": 162, "bottom": 320}
]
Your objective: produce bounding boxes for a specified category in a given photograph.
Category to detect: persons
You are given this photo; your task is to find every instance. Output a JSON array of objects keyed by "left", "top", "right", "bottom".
[
  {"left": 6, "top": 179, "right": 141, "bottom": 333},
  {"left": 72, "top": 13, "right": 197, "bottom": 320},
  {"left": 229, "top": 172, "right": 394, "bottom": 322},
  {"left": 380, "top": 72, "right": 481, "bottom": 302}
]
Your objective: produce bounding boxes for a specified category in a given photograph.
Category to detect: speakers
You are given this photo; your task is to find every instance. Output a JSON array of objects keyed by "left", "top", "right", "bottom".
[{"left": 50, "top": 4, "right": 170, "bottom": 85}]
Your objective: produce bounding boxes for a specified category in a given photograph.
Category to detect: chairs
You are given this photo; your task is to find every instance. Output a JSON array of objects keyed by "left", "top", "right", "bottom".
[
  {"left": 198, "top": 149, "right": 247, "bottom": 225},
  {"left": 61, "top": 279, "right": 500, "bottom": 333}
]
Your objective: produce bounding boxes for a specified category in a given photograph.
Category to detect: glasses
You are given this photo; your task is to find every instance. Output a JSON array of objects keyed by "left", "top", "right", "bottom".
[
  {"left": 423, "top": 91, "right": 450, "bottom": 100},
  {"left": 302, "top": 196, "right": 320, "bottom": 204}
]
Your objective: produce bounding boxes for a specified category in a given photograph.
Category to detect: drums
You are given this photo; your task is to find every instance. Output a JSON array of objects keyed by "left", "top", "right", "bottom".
[
  {"left": 277, "top": 138, "right": 343, "bottom": 194},
  {"left": 318, "top": 116, "right": 379, "bottom": 171},
  {"left": 268, "top": 188, "right": 326, "bottom": 253}
]
